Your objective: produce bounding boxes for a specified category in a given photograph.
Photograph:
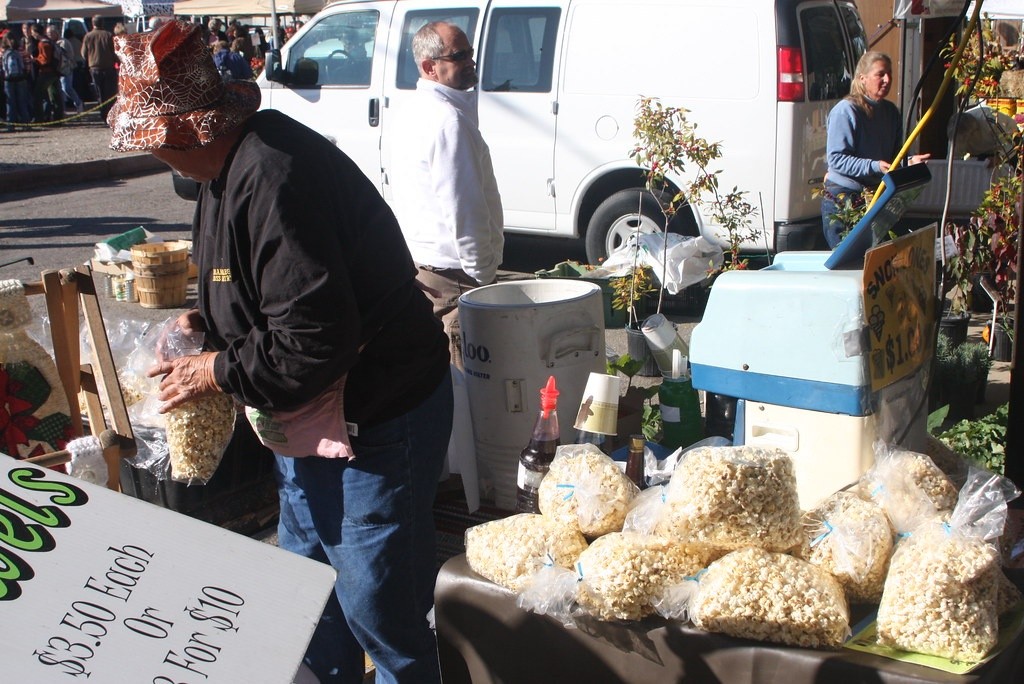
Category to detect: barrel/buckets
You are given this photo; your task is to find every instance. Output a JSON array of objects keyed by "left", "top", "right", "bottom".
[
  {"left": 458, "top": 278, "right": 607, "bottom": 510},
  {"left": 131, "top": 243, "right": 189, "bottom": 308}
]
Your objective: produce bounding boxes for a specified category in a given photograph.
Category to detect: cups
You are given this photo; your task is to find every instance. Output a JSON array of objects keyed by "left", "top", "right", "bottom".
[
  {"left": 573, "top": 372, "right": 620, "bottom": 436},
  {"left": 641, "top": 313, "right": 689, "bottom": 379}
]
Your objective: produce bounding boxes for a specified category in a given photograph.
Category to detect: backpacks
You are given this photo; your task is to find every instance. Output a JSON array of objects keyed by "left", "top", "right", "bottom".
[
  {"left": 2, "top": 49, "right": 25, "bottom": 80},
  {"left": 42, "top": 39, "right": 73, "bottom": 75}
]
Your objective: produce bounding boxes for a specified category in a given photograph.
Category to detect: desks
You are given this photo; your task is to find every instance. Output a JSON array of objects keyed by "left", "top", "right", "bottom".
[{"left": 434, "top": 537, "right": 1024, "bottom": 684}]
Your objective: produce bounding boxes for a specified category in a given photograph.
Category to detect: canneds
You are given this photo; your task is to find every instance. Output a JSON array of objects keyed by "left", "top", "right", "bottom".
[{"left": 114, "top": 281, "right": 127, "bottom": 302}]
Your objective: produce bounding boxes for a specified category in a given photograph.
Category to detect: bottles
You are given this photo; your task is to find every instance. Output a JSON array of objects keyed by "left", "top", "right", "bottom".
[
  {"left": 574, "top": 428, "right": 613, "bottom": 458},
  {"left": 624, "top": 435, "right": 648, "bottom": 491},
  {"left": 516, "top": 376, "right": 561, "bottom": 514}
]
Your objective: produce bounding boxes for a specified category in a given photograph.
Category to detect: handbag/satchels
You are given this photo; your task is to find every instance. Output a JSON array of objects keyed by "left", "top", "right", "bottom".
[{"left": 245, "top": 375, "right": 353, "bottom": 458}]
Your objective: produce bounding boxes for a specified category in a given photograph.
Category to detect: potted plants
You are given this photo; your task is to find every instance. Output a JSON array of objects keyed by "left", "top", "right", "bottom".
[
  {"left": 943, "top": 109, "right": 1024, "bottom": 363},
  {"left": 938, "top": 9, "right": 1024, "bottom": 319},
  {"left": 930, "top": 331, "right": 956, "bottom": 399},
  {"left": 611, "top": 92, "right": 771, "bottom": 437},
  {"left": 939, "top": 214, "right": 991, "bottom": 346},
  {"left": 946, "top": 327, "right": 993, "bottom": 407}
]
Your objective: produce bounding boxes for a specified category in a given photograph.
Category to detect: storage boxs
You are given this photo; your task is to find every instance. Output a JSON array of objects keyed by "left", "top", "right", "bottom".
[{"left": 89, "top": 257, "right": 198, "bottom": 279}]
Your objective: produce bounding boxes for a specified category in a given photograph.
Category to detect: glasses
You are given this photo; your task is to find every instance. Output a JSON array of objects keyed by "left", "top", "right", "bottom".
[{"left": 430, "top": 49, "right": 474, "bottom": 60}]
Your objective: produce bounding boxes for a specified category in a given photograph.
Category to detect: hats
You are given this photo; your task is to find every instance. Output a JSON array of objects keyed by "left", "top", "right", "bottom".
[{"left": 106, "top": 19, "right": 261, "bottom": 151}]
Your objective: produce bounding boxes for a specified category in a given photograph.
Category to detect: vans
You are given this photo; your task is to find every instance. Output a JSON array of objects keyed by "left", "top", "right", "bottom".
[{"left": 173, "top": 0, "right": 871, "bottom": 315}]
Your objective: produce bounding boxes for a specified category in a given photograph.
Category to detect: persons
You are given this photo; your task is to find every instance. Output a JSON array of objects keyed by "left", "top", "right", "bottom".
[
  {"left": 381, "top": 21, "right": 504, "bottom": 506},
  {"left": 0, "top": 15, "right": 305, "bottom": 131},
  {"left": 822, "top": 51, "right": 931, "bottom": 250},
  {"left": 106, "top": 21, "right": 455, "bottom": 684}
]
